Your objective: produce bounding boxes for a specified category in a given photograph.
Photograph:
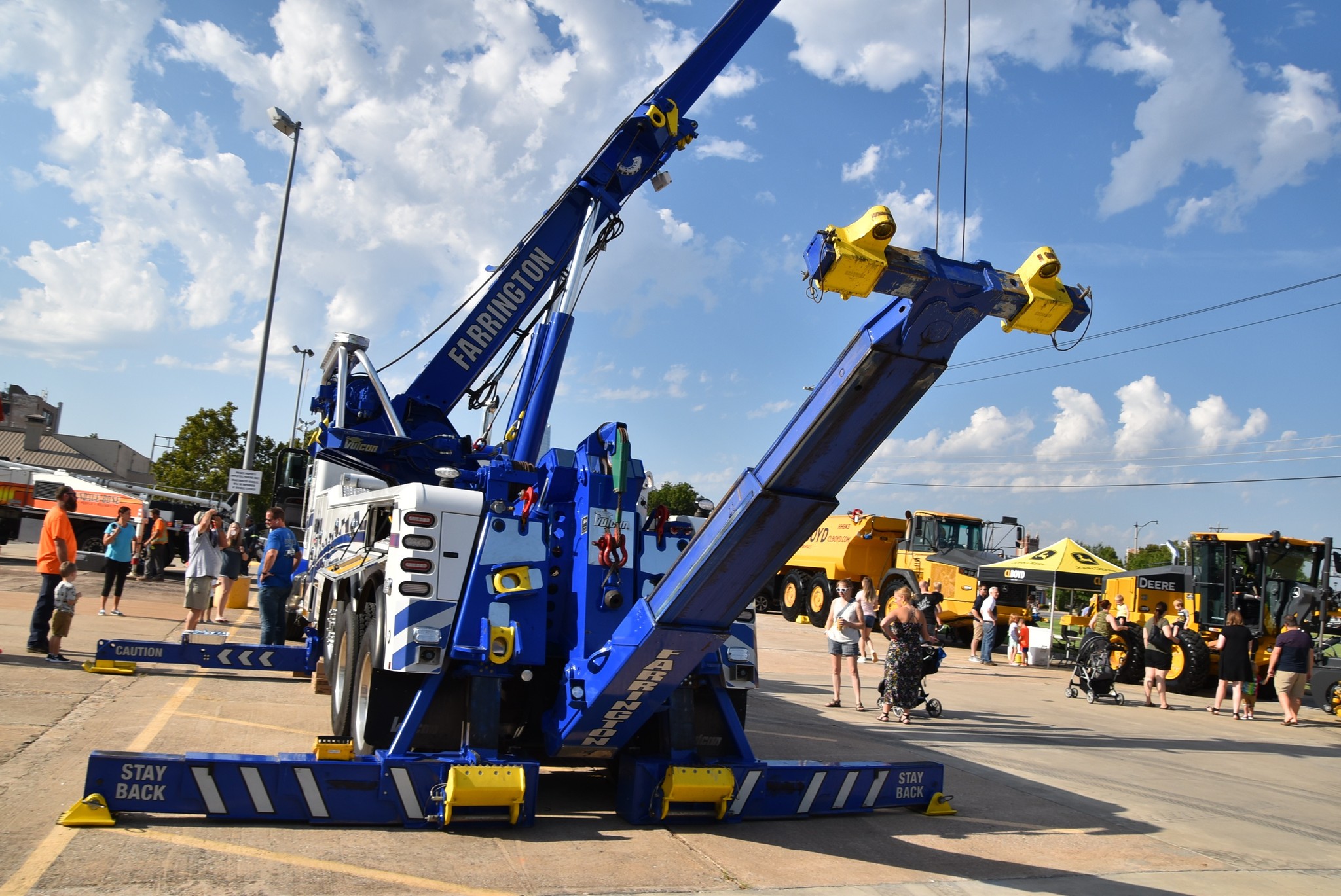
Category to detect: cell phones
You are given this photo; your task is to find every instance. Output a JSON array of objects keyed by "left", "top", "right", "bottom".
[{"left": 111, "top": 523, "right": 117, "bottom": 529}]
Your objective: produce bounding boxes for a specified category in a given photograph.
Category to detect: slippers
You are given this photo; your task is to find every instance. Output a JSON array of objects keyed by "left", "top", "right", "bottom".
[
  {"left": 1159, "top": 705, "right": 1174, "bottom": 710},
  {"left": 1280, "top": 720, "right": 1298, "bottom": 725},
  {"left": 1144, "top": 702, "right": 1156, "bottom": 707}
]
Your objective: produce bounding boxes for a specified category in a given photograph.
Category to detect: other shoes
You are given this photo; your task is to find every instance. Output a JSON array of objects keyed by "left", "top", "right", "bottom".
[
  {"left": 27, "top": 646, "right": 47, "bottom": 654},
  {"left": 938, "top": 624, "right": 944, "bottom": 631},
  {"left": 871, "top": 650, "right": 878, "bottom": 663},
  {"left": 1009, "top": 661, "right": 1020, "bottom": 666},
  {"left": 216, "top": 618, "right": 227, "bottom": 623},
  {"left": 199, "top": 619, "right": 214, "bottom": 624},
  {"left": 980, "top": 661, "right": 998, "bottom": 666},
  {"left": 857, "top": 657, "right": 867, "bottom": 663},
  {"left": 1240, "top": 715, "right": 1254, "bottom": 720},
  {"left": 1020, "top": 663, "right": 1029, "bottom": 666}
]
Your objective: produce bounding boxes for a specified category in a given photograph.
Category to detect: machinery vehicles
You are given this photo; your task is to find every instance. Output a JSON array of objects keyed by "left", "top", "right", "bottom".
[
  {"left": 54, "top": 0, "right": 1093, "bottom": 828},
  {"left": 1059, "top": 529, "right": 1341, "bottom": 720}
]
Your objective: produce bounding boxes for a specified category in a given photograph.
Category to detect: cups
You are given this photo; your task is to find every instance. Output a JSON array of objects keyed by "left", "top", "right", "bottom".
[{"left": 836, "top": 616, "right": 843, "bottom": 631}]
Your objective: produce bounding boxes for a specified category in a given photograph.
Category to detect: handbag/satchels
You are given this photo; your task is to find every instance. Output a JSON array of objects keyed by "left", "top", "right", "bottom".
[{"left": 1148, "top": 624, "right": 1172, "bottom": 652}]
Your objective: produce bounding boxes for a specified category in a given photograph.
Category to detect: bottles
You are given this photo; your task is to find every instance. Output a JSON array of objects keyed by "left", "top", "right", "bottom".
[{"left": 231, "top": 530, "right": 237, "bottom": 535}]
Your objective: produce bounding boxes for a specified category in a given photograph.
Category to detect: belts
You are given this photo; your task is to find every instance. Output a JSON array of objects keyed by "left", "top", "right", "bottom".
[{"left": 984, "top": 621, "right": 993, "bottom": 622}]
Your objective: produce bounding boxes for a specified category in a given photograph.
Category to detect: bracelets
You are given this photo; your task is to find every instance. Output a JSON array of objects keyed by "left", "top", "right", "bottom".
[
  {"left": 241, "top": 552, "right": 245, "bottom": 554},
  {"left": 824, "top": 631, "right": 828, "bottom": 634},
  {"left": 262, "top": 572, "right": 267, "bottom": 575},
  {"left": 218, "top": 527, "right": 223, "bottom": 531}
]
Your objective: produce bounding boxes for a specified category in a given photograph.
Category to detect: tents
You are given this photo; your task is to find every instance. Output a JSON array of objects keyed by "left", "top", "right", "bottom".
[{"left": 976, "top": 538, "right": 1127, "bottom": 669}]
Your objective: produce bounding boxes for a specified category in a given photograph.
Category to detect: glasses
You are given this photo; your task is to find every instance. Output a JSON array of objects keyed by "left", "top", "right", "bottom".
[
  {"left": 980, "top": 588, "right": 988, "bottom": 591},
  {"left": 836, "top": 587, "right": 851, "bottom": 592},
  {"left": 265, "top": 518, "right": 277, "bottom": 521},
  {"left": 64, "top": 492, "right": 76, "bottom": 497}
]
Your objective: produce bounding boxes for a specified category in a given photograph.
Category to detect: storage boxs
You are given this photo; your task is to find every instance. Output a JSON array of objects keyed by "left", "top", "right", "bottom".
[{"left": 1007, "top": 626, "right": 1052, "bottom": 668}]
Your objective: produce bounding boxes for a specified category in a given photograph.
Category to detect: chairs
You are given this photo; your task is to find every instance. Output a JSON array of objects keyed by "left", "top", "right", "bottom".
[{"left": 1053, "top": 629, "right": 1079, "bottom": 668}]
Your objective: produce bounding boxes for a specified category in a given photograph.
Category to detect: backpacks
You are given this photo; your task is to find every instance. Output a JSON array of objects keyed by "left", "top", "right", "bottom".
[{"left": 1090, "top": 651, "right": 1113, "bottom": 680}]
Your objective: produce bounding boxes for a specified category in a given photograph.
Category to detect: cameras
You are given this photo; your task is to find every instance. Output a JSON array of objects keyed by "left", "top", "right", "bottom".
[{"left": 212, "top": 513, "right": 225, "bottom": 519}]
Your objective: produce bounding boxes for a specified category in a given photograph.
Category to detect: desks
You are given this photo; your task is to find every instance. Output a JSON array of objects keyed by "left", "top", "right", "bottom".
[{"left": 1052, "top": 634, "right": 1083, "bottom": 669}]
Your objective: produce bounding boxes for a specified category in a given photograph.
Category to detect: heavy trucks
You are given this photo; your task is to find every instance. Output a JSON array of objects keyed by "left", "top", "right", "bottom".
[
  {"left": 756, "top": 509, "right": 1051, "bottom": 648},
  {"left": 0, "top": 456, "right": 234, "bottom": 576}
]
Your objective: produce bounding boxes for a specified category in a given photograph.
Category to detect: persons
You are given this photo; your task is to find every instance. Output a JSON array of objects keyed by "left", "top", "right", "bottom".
[
  {"left": 198, "top": 578, "right": 221, "bottom": 624},
  {"left": 1113, "top": 594, "right": 1130, "bottom": 626},
  {"left": 46, "top": 561, "right": 82, "bottom": 663},
  {"left": 824, "top": 578, "right": 865, "bottom": 711},
  {"left": 98, "top": 506, "right": 137, "bottom": 615},
  {"left": 1172, "top": 598, "right": 1190, "bottom": 637},
  {"left": 256, "top": 506, "right": 301, "bottom": 644},
  {"left": 183, "top": 510, "right": 228, "bottom": 640},
  {"left": 968, "top": 584, "right": 989, "bottom": 662},
  {"left": 854, "top": 576, "right": 878, "bottom": 663},
  {"left": 911, "top": 580, "right": 944, "bottom": 645},
  {"left": 980, "top": 587, "right": 999, "bottom": 666},
  {"left": 1267, "top": 615, "right": 1314, "bottom": 726},
  {"left": 216, "top": 521, "right": 252, "bottom": 622},
  {"left": 240, "top": 513, "right": 258, "bottom": 576},
  {"left": 876, "top": 585, "right": 939, "bottom": 724},
  {"left": 1089, "top": 600, "right": 1129, "bottom": 666},
  {"left": 1017, "top": 618, "right": 1029, "bottom": 667},
  {"left": 1008, "top": 613, "right": 1021, "bottom": 666},
  {"left": 1142, "top": 601, "right": 1179, "bottom": 709},
  {"left": 1206, "top": 609, "right": 1269, "bottom": 720},
  {"left": 1026, "top": 595, "right": 1040, "bottom": 628},
  {"left": 136, "top": 508, "right": 168, "bottom": 582},
  {"left": 25, "top": 485, "right": 78, "bottom": 654}
]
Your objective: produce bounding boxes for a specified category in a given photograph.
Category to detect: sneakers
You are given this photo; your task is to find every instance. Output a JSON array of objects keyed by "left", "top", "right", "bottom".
[
  {"left": 46, "top": 654, "right": 72, "bottom": 663},
  {"left": 98, "top": 609, "right": 123, "bottom": 616},
  {"left": 968, "top": 656, "right": 981, "bottom": 662}
]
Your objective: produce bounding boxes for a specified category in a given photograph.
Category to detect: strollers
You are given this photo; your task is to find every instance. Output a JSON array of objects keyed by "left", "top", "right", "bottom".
[
  {"left": 877, "top": 639, "right": 945, "bottom": 717},
  {"left": 1064, "top": 631, "right": 1128, "bottom": 706}
]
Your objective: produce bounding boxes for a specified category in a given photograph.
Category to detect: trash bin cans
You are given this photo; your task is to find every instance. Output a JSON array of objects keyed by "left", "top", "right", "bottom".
[{"left": 1014, "top": 652, "right": 1022, "bottom": 663}]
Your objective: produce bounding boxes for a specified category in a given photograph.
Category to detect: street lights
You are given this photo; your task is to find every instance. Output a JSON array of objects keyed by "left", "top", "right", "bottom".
[
  {"left": 234, "top": 105, "right": 301, "bottom": 544},
  {"left": 1160, "top": 543, "right": 1187, "bottom": 566},
  {"left": 1133, "top": 520, "right": 1160, "bottom": 555},
  {"left": 284, "top": 345, "right": 315, "bottom": 486}
]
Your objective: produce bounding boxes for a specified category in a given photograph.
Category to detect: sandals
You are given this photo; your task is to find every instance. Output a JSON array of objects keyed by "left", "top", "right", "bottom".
[
  {"left": 876, "top": 712, "right": 889, "bottom": 722},
  {"left": 825, "top": 699, "right": 841, "bottom": 707},
  {"left": 1205, "top": 705, "right": 1220, "bottom": 715},
  {"left": 899, "top": 714, "right": 911, "bottom": 725},
  {"left": 856, "top": 703, "right": 864, "bottom": 712},
  {"left": 1233, "top": 713, "right": 1239, "bottom": 720}
]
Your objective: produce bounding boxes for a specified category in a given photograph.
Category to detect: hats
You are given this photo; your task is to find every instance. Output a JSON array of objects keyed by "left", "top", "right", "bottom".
[
  {"left": 194, "top": 511, "right": 205, "bottom": 523},
  {"left": 245, "top": 514, "right": 254, "bottom": 522}
]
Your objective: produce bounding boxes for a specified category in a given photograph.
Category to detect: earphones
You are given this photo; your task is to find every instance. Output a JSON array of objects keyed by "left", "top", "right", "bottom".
[{"left": 276, "top": 520, "right": 279, "bottom": 524}]
